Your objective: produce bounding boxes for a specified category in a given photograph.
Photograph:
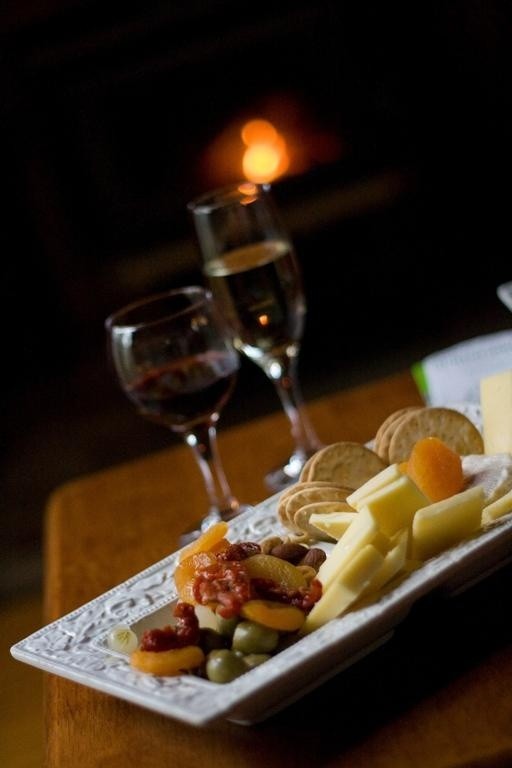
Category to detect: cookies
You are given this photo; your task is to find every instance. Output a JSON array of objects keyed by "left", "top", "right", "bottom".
[{"left": 276, "top": 405, "right": 484, "bottom": 541}]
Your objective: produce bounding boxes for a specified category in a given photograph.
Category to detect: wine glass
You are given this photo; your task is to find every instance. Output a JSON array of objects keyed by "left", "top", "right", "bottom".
[
  {"left": 104, "top": 286, "right": 256, "bottom": 543},
  {"left": 185, "top": 184, "right": 354, "bottom": 490}
]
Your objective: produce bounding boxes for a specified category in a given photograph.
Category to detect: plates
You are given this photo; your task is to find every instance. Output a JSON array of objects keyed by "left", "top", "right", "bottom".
[{"left": 9, "top": 436, "right": 512, "bottom": 730}]
